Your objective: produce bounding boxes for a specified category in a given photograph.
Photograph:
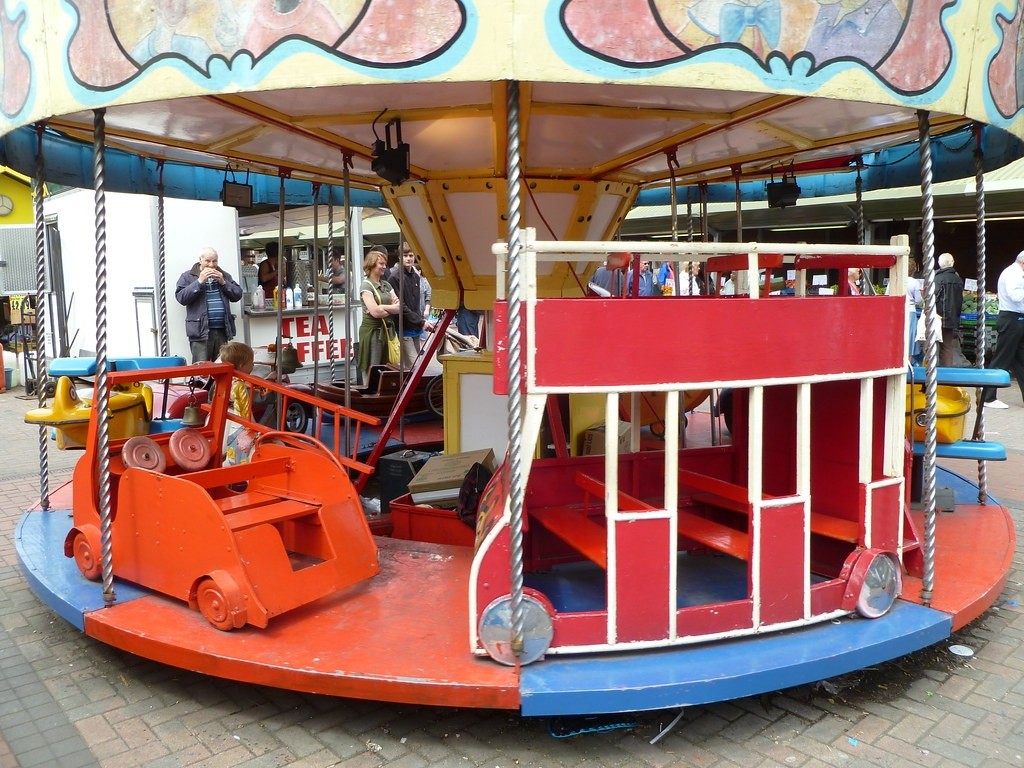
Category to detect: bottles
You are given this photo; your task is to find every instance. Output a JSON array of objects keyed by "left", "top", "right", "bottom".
[{"left": 252, "top": 283, "right": 315, "bottom": 309}]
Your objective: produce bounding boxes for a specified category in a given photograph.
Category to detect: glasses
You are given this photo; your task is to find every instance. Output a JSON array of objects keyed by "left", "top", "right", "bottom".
[
  {"left": 858, "top": 271, "right": 861, "bottom": 274},
  {"left": 641, "top": 263, "right": 649, "bottom": 266}
]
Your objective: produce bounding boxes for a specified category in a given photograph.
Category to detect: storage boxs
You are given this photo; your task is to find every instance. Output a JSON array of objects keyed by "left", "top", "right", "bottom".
[
  {"left": 405, "top": 448, "right": 496, "bottom": 509},
  {"left": 582, "top": 419, "right": 633, "bottom": 456}
]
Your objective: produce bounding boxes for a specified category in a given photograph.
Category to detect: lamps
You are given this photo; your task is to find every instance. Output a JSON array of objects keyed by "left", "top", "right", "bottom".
[
  {"left": 220, "top": 162, "right": 253, "bottom": 209},
  {"left": 372, "top": 115, "right": 411, "bottom": 185},
  {"left": 766, "top": 176, "right": 802, "bottom": 209}
]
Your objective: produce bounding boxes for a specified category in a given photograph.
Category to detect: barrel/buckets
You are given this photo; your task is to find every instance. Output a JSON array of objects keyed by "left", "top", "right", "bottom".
[{"left": 4, "top": 368, "right": 14, "bottom": 389}]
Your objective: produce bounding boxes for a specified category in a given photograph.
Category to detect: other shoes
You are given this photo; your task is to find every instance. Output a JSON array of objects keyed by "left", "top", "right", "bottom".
[{"left": 983, "top": 399, "right": 1009, "bottom": 409}]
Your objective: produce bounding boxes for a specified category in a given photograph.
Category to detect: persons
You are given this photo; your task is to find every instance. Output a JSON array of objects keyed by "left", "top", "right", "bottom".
[
  {"left": 355, "top": 251, "right": 400, "bottom": 386},
  {"left": 848, "top": 267, "right": 861, "bottom": 295},
  {"left": 591, "top": 252, "right": 737, "bottom": 296},
  {"left": 983, "top": 250, "right": 1024, "bottom": 409},
  {"left": 885, "top": 259, "right": 924, "bottom": 362},
  {"left": 935, "top": 253, "right": 964, "bottom": 367},
  {"left": 316, "top": 245, "right": 432, "bottom": 355},
  {"left": 175, "top": 248, "right": 243, "bottom": 365},
  {"left": 387, "top": 242, "right": 436, "bottom": 372},
  {"left": 258, "top": 242, "right": 285, "bottom": 298},
  {"left": 219, "top": 342, "right": 255, "bottom": 422}
]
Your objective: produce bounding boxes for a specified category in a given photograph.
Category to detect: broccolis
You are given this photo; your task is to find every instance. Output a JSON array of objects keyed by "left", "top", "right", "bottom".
[{"left": 961, "top": 294, "right": 999, "bottom": 314}]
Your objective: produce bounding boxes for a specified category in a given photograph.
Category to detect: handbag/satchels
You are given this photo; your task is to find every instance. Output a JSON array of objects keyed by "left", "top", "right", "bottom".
[
  {"left": 387, "top": 333, "right": 401, "bottom": 365},
  {"left": 915, "top": 301, "right": 944, "bottom": 344}
]
